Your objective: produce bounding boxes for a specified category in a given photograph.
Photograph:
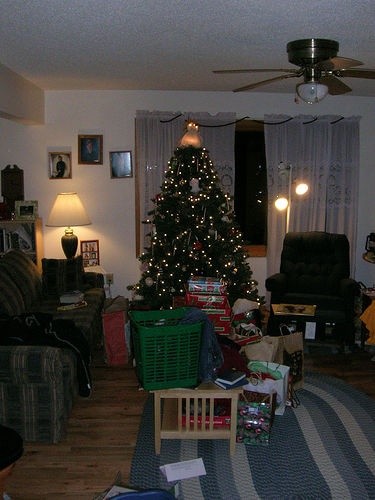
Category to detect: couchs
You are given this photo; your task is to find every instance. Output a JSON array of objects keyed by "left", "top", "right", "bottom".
[
  {"left": 0, "top": 251, "right": 106, "bottom": 444},
  {"left": 265, "top": 231, "right": 362, "bottom": 348}
]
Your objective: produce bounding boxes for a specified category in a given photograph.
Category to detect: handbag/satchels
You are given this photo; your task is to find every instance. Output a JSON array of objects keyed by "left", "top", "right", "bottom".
[{"left": 236, "top": 323, "right": 304, "bottom": 446}]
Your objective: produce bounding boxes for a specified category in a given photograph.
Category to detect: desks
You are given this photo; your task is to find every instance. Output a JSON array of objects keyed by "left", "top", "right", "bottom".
[{"left": 148, "top": 387, "right": 240, "bottom": 456}]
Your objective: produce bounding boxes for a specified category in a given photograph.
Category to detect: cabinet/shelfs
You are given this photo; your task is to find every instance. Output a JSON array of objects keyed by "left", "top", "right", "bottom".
[{"left": 0, "top": 216, "right": 43, "bottom": 276}]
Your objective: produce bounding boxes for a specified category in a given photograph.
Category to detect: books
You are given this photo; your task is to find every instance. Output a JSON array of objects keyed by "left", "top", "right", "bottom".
[
  {"left": 271, "top": 303, "right": 315, "bottom": 316},
  {"left": 60, "top": 291, "right": 84, "bottom": 303},
  {"left": 214, "top": 367, "right": 249, "bottom": 390}
]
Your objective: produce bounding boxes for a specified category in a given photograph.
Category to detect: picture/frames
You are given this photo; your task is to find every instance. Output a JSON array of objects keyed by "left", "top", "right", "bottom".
[
  {"left": 109, "top": 150, "right": 133, "bottom": 179},
  {"left": 78, "top": 135, "right": 103, "bottom": 165},
  {"left": 15, "top": 201, "right": 38, "bottom": 221},
  {"left": 48, "top": 152, "right": 73, "bottom": 180}
]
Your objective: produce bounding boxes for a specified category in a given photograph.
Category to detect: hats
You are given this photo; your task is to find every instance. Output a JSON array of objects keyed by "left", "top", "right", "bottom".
[{"left": 0, "top": 425, "right": 24, "bottom": 471}]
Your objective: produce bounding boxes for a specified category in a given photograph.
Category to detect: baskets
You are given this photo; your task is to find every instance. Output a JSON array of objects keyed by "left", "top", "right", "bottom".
[{"left": 128, "top": 309, "right": 202, "bottom": 392}]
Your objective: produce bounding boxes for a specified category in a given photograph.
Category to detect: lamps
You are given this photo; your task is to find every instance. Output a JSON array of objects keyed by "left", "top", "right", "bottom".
[
  {"left": 45, "top": 192, "right": 92, "bottom": 258},
  {"left": 295, "top": 82, "right": 329, "bottom": 105}
]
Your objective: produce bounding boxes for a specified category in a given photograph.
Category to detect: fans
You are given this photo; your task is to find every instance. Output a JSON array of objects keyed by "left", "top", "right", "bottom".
[{"left": 212, "top": 39, "right": 375, "bottom": 95}]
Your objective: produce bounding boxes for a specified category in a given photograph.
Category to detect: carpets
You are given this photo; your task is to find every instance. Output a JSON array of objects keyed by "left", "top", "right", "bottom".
[{"left": 129, "top": 374, "right": 375, "bottom": 500}]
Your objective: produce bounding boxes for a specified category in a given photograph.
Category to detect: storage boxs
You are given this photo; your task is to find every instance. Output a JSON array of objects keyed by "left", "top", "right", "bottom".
[
  {"left": 128, "top": 273, "right": 305, "bottom": 446},
  {"left": 101, "top": 297, "right": 132, "bottom": 368}
]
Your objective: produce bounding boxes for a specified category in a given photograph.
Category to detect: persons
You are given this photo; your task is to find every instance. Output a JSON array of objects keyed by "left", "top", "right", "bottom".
[
  {"left": 56, "top": 156, "right": 65, "bottom": 177},
  {"left": 85, "top": 140, "right": 97, "bottom": 160}
]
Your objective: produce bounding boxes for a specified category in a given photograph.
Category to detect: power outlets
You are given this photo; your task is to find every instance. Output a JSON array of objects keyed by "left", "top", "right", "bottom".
[{"left": 106, "top": 274, "right": 114, "bottom": 285}]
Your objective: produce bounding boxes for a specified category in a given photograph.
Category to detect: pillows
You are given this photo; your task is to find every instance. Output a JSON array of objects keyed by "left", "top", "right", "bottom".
[{"left": 41, "top": 258, "right": 86, "bottom": 289}]
